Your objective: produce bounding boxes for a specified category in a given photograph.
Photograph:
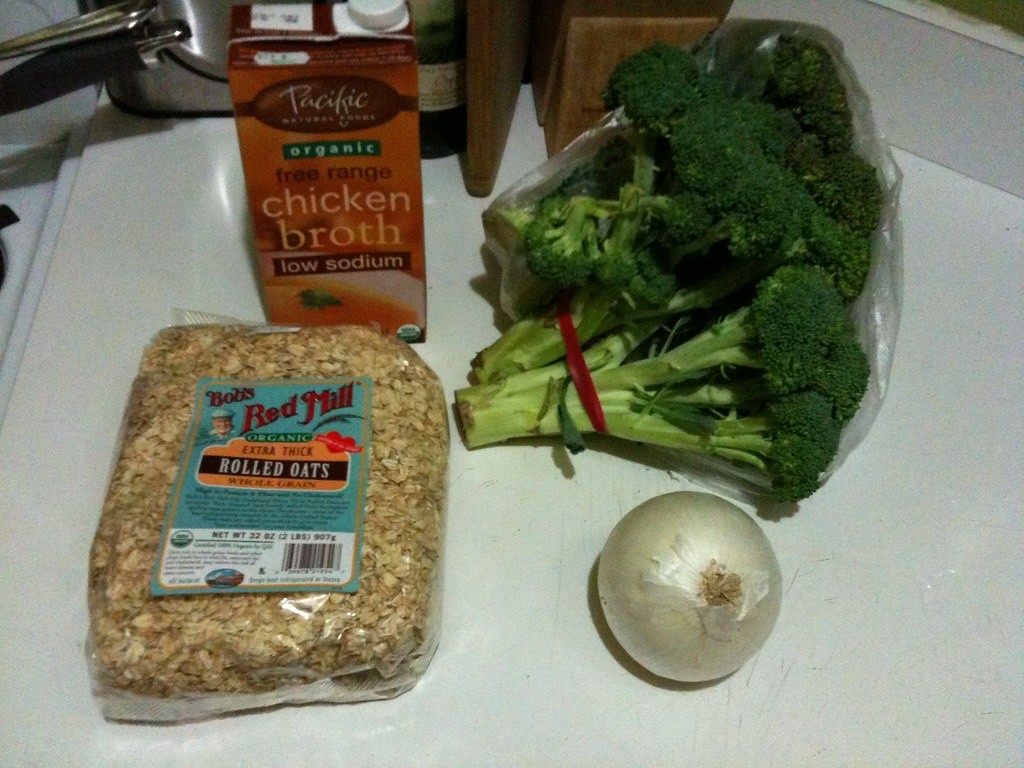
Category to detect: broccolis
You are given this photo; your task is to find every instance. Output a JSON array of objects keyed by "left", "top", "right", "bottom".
[{"left": 454, "top": 32, "right": 885, "bottom": 498}]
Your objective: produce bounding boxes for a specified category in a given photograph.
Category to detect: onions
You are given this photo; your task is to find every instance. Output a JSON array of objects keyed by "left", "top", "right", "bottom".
[{"left": 597, "top": 491, "right": 782, "bottom": 684}]
